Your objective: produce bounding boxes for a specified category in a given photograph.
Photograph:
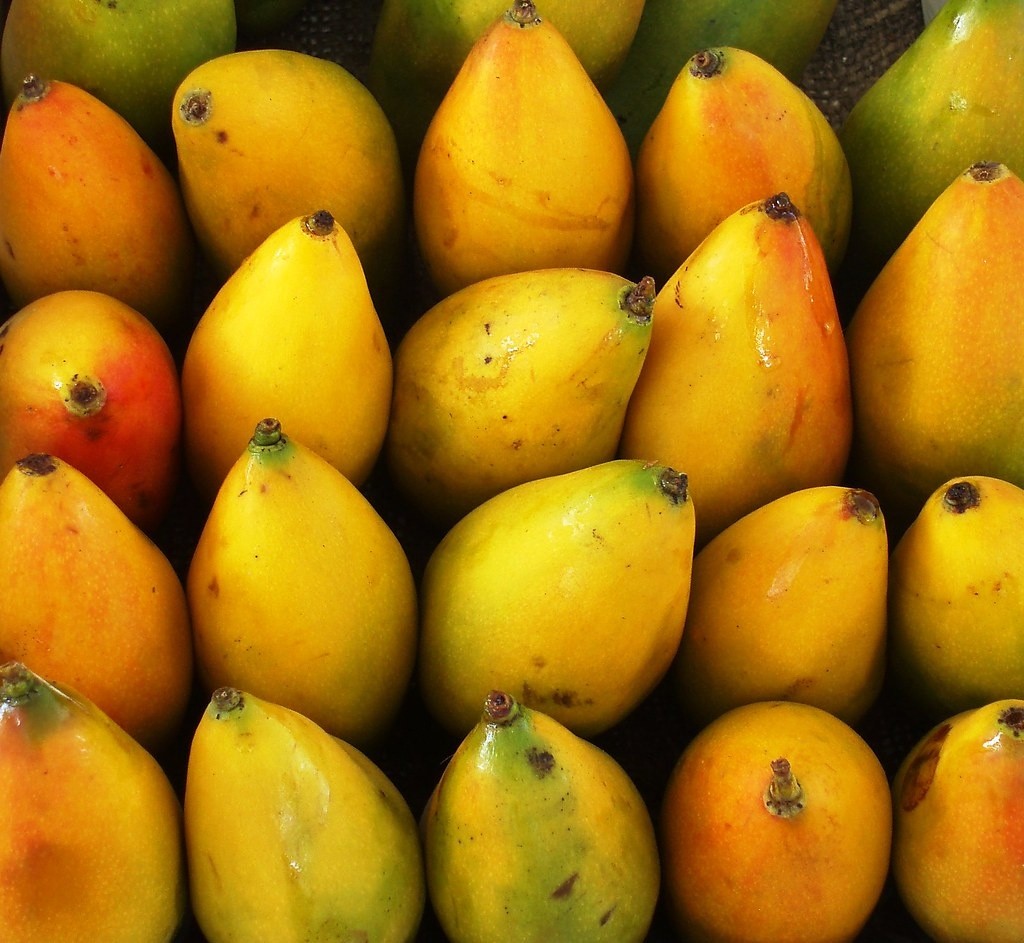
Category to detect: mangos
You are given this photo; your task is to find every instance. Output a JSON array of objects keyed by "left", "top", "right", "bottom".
[{"left": 0, "top": 0, "right": 1024, "bottom": 943}]
